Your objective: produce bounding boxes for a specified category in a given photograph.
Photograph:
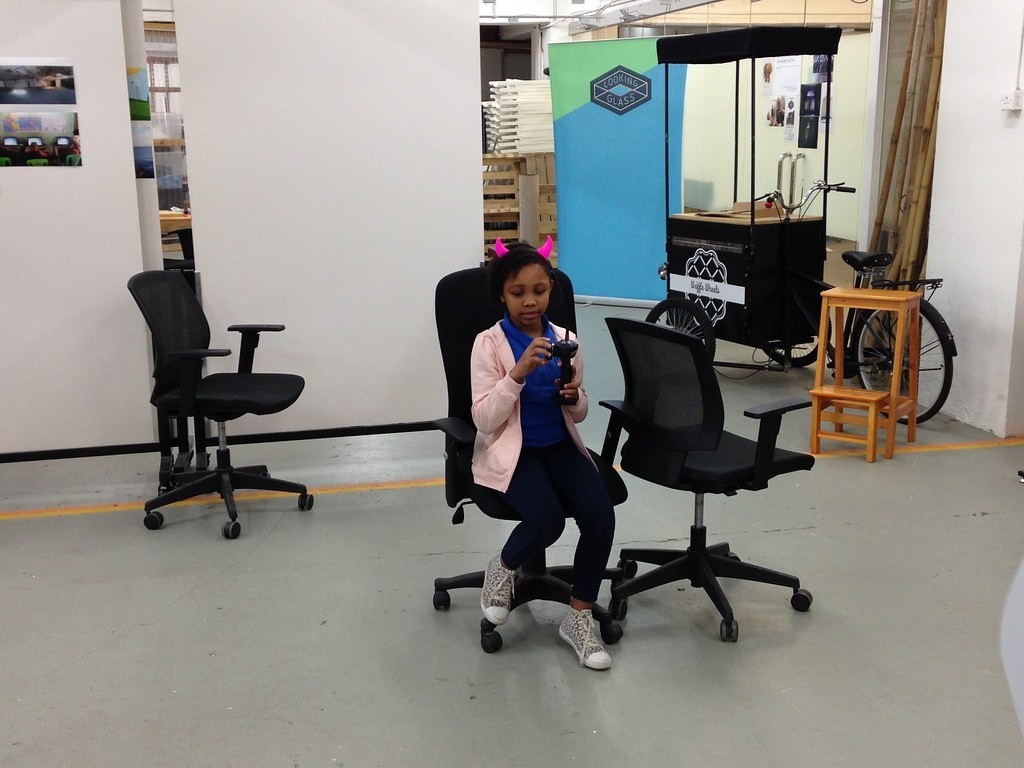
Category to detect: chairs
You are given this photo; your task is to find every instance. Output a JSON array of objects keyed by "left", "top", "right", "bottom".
[
  {"left": 605, "top": 318, "right": 815, "bottom": 642},
  {"left": 126, "top": 271, "right": 313, "bottom": 536},
  {"left": 434, "top": 267, "right": 627, "bottom": 653}
]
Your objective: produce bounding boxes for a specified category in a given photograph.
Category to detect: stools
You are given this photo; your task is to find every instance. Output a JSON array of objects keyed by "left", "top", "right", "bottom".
[{"left": 809, "top": 285, "right": 923, "bottom": 463}]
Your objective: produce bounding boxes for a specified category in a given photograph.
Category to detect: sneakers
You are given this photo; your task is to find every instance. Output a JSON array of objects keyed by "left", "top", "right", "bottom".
[
  {"left": 480, "top": 553, "right": 517, "bottom": 625},
  {"left": 559, "top": 602, "right": 612, "bottom": 670}
]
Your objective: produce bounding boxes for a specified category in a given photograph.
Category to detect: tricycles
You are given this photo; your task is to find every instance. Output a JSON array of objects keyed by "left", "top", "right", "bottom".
[{"left": 645, "top": 25, "right": 958, "bottom": 426}]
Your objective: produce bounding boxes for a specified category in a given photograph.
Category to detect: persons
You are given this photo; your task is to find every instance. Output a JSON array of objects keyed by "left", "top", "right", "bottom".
[
  {"left": 767, "top": 98, "right": 784, "bottom": 127},
  {"left": 470, "top": 243, "right": 616, "bottom": 669},
  {"left": 25, "top": 128, "right": 81, "bottom": 159}
]
